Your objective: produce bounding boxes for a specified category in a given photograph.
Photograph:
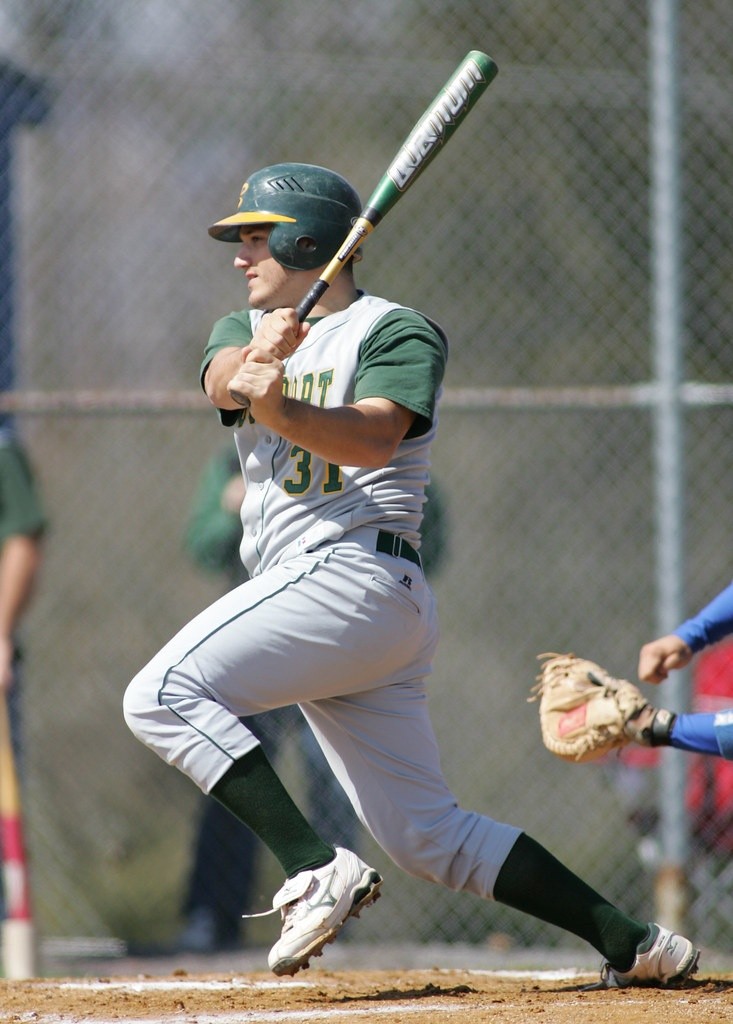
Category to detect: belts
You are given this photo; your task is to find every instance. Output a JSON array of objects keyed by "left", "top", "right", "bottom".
[{"left": 377, "top": 528, "right": 421, "bottom": 564}]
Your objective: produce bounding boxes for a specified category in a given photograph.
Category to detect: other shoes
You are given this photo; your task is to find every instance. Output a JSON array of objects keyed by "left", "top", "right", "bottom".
[{"left": 178, "top": 906, "right": 235, "bottom": 953}]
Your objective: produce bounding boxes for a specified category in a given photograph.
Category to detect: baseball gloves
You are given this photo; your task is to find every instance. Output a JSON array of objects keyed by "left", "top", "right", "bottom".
[{"left": 526, "top": 651, "right": 650, "bottom": 763}]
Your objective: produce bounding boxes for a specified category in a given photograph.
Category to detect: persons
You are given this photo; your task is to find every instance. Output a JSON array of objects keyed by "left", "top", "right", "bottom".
[
  {"left": 1, "top": 413, "right": 47, "bottom": 693},
  {"left": 130, "top": 434, "right": 450, "bottom": 956},
  {"left": 122, "top": 163, "right": 704, "bottom": 990},
  {"left": 538, "top": 579, "right": 733, "bottom": 925}
]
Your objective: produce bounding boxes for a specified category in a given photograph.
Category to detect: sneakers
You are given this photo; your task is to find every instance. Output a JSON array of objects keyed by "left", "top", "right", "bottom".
[
  {"left": 243, "top": 843, "right": 382, "bottom": 978},
  {"left": 600, "top": 922, "right": 702, "bottom": 989}
]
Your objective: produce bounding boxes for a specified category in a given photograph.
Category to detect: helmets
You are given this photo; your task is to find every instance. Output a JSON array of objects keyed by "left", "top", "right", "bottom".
[{"left": 207, "top": 162, "right": 361, "bottom": 272}]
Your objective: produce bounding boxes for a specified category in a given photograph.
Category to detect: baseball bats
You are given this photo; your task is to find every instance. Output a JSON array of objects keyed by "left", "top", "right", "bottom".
[
  {"left": 226, "top": 46, "right": 501, "bottom": 407},
  {"left": 1, "top": 690, "right": 39, "bottom": 984}
]
